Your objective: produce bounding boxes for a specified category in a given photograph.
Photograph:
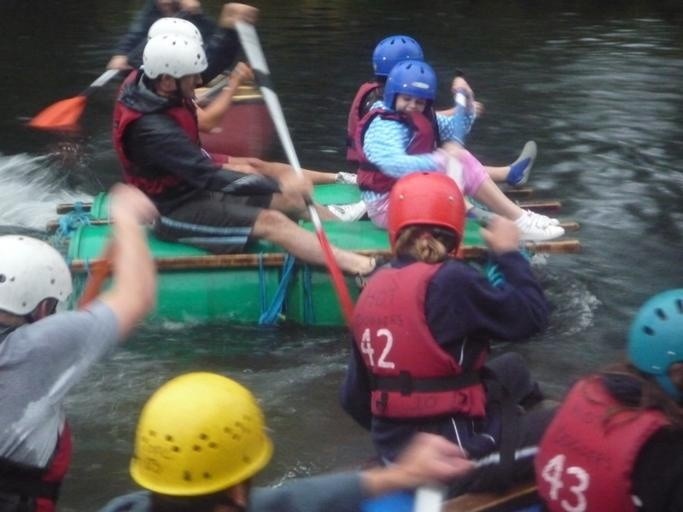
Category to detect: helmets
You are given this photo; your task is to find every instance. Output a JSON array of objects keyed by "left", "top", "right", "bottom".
[
  {"left": 129, "top": 370, "right": 273, "bottom": 498},
  {"left": 624, "top": 289, "right": 682, "bottom": 395},
  {"left": 143, "top": 16, "right": 209, "bottom": 79},
  {"left": 388, "top": 171, "right": 466, "bottom": 258},
  {"left": 371, "top": 35, "right": 424, "bottom": 76},
  {"left": 0, "top": 234, "right": 74, "bottom": 316},
  {"left": 382, "top": 59, "right": 438, "bottom": 111}
]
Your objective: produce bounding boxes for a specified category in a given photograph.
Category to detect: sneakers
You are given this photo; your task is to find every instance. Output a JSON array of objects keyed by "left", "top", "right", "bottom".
[
  {"left": 335, "top": 171, "right": 358, "bottom": 185},
  {"left": 509, "top": 140, "right": 537, "bottom": 188},
  {"left": 328, "top": 199, "right": 367, "bottom": 223},
  {"left": 510, "top": 209, "right": 565, "bottom": 241}
]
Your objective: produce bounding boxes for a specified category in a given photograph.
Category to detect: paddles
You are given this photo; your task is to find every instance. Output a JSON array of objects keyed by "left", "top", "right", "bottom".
[{"left": 26, "top": 69, "right": 119, "bottom": 129}]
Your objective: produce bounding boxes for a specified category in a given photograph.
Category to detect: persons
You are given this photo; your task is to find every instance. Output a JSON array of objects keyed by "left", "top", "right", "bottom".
[
  {"left": 339, "top": 35, "right": 562, "bottom": 497},
  {"left": 104, "top": 369, "right": 477, "bottom": 510},
  {"left": 106, "top": 1, "right": 384, "bottom": 276},
  {"left": 532, "top": 282, "right": 682, "bottom": 511},
  {"left": 0, "top": 178, "right": 157, "bottom": 510}
]
